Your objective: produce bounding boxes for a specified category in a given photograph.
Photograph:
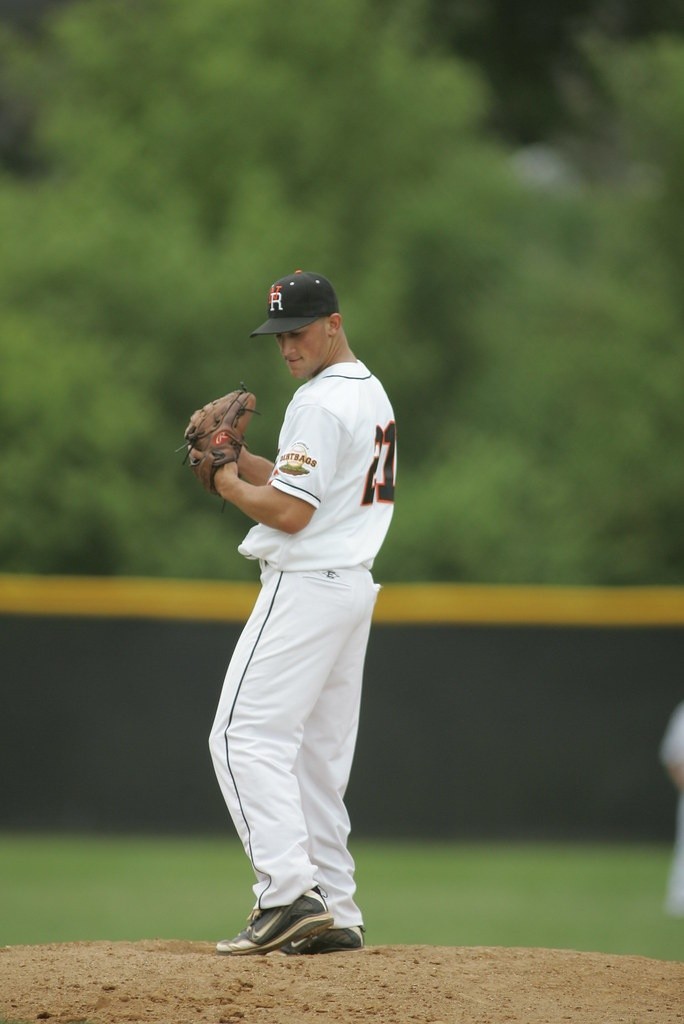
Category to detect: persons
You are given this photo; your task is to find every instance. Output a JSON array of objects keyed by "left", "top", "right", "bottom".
[{"left": 183, "top": 271, "right": 396, "bottom": 955}]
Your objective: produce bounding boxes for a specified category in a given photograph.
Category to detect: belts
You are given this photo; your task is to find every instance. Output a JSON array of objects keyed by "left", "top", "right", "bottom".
[{"left": 265, "top": 560, "right": 268, "bottom": 566}]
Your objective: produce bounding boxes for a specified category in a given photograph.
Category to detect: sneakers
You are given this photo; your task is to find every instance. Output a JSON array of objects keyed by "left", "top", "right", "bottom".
[
  {"left": 280, "top": 925, "right": 366, "bottom": 954},
  {"left": 216, "top": 885, "right": 334, "bottom": 956}
]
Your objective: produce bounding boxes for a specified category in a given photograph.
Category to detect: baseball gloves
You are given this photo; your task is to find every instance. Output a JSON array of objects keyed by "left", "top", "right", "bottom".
[{"left": 175, "top": 380, "right": 262, "bottom": 510}]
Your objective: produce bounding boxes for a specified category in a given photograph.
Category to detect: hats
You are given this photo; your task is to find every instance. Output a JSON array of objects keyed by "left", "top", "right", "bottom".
[{"left": 249, "top": 269, "right": 339, "bottom": 338}]
